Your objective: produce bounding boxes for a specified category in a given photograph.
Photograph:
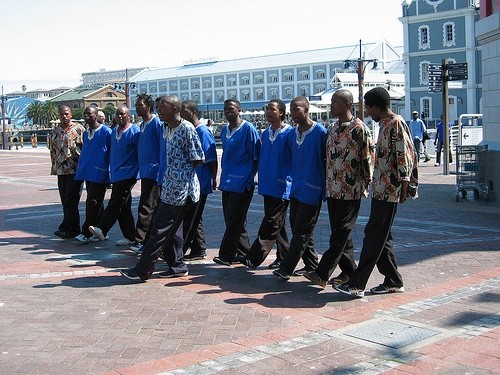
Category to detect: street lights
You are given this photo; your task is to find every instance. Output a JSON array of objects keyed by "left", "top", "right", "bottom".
[
  {"left": 113, "top": 68, "right": 138, "bottom": 110},
  {"left": 342, "top": 38, "right": 378, "bottom": 122}
]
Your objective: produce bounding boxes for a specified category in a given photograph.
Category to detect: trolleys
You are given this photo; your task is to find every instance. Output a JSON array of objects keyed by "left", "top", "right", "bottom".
[{"left": 455, "top": 112, "right": 490, "bottom": 203}]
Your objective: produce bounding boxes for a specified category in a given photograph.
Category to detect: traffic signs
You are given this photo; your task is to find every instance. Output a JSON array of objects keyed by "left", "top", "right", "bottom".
[
  {"left": 427, "top": 63, "right": 443, "bottom": 94},
  {"left": 447, "top": 62, "right": 468, "bottom": 81}
]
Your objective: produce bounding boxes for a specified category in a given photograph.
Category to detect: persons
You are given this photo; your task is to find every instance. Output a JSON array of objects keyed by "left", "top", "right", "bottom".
[
  {"left": 333, "top": 87, "right": 420, "bottom": 297},
  {"left": 89, "top": 106, "right": 139, "bottom": 244},
  {"left": 73, "top": 106, "right": 111, "bottom": 241},
  {"left": 131, "top": 93, "right": 161, "bottom": 252},
  {"left": 273, "top": 96, "right": 327, "bottom": 280},
  {"left": 154, "top": 96, "right": 183, "bottom": 259},
  {"left": 97, "top": 111, "right": 105, "bottom": 125},
  {"left": 304, "top": 89, "right": 374, "bottom": 289},
  {"left": 409, "top": 111, "right": 429, "bottom": 162},
  {"left": 240, "top": 98, "right": 292, "bottom": 268},
  {"left": 213, "top": 99, "right": 261, "bottom": 266},
  {"left": 433, "top": 114, "right": 454, "bottom": 166},
  {"left": 120, "top": 95, "right": 205, "bottom": 281},
  {"left": 180, "top": 100, "right": 218, "bottom": 261},
  {"left": 49, "top": 105, "right": 85, "bottom": 238}
]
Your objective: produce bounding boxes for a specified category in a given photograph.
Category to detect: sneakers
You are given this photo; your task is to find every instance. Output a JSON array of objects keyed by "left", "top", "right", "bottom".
[
  {"left": 331, "top": 283, "right": 364, "bottom": 297},
  {"left": 370, "top": 284, "right": 404, "bottom": 293}
]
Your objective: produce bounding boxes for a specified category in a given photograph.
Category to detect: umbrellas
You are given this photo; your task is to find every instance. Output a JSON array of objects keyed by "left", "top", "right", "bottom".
[{"left": 238, "top": 85, "right": 405, "bottom": 115}]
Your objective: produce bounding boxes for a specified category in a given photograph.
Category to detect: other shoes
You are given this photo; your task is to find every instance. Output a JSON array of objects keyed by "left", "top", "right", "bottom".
[
  {"left": 422, "top": 158, "right": 430, "bottom": 162},
  {"left": 269, "top": 259, "right": 282, "bottom": 269},
  {"left": 331, "top": 273, "right": 349, "bottom": 283},
  {"left": 116, "top": 239, "right": 135, "bottom": 245},
  {"left": 294, "top": 265, "right": 315, "bottom": 275},
  {"left": 89, "top": 234, "right": 109, "bottom": 241},
  {"left": 158, "top": 269, "right": 188, "bottom": 277},
  {"left": 121, "top": 268, "right": 148, "bottom": 280},
  {"left": 54, "top": 229, "right": 81, "bottom": 239},
  {"left": 89, "top": 226, "right": 104, "bottom": 240},
  {"left": 159, "top": 253, "right": 165, "bottom": 260},
  {"left": 231, "top": 256, "right": 242, "bottom": 264},
  {"left": 237, "top": 253, "right": 257, "bottom": 269},
  {"left": 212, "top": 257, "right": 231, "bottom": 266},
  {"left": 130, "top": 243, "right": 143, "bottom": 253},
  {"left": 449, "top": 163, "right": 452, "bottom": 165},
  {"left": 75, "top": 234, "right": 88, "bottom": 242},
  {"left": 303, "top": 270, "right": 326, "bottom": 288},
  {"left": 273, "top": 268, "right": 292, "bottom": 279},
  {"left": 184, "top": 253, "right": 207, "bottom": 260},
  {"left": 434, "top": 163, "right": 440, "bottom": 166}
]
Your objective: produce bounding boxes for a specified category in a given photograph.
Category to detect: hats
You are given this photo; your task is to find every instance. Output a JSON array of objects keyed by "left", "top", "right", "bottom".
[{"left": 412, "top": 111, "right": 418, "bottom": 114}]
